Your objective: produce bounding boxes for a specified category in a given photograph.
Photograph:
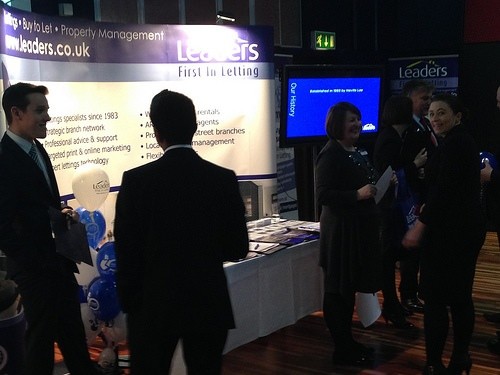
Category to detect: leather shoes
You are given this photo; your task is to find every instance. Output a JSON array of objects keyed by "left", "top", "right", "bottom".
[
  {"left": 401, "top": 293, "right": 424, "bottom": 312},
  {"left": 333, "top": 345, "right": 377, "bottom": 367}
]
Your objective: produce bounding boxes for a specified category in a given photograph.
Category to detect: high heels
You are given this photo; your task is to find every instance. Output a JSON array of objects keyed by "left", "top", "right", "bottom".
[
  {"left": 423, "top": 363, "right": 448, "bottom": 375},
  {"left": 381, "top": 300, "right": 416, "bottom": 329},
  {"left": 448, "top": 352, "right": 474, "bottom": 375}
]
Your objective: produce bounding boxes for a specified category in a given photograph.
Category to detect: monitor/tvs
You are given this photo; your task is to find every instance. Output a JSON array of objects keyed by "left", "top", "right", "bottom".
[{"left": 280, "top": 68, "right": 388, "bottom": 149}]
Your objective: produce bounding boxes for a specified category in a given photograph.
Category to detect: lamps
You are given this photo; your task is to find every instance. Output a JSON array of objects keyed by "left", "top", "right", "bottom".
[{"left": 215, "top": 11, "right": 236, "bottom": 24}]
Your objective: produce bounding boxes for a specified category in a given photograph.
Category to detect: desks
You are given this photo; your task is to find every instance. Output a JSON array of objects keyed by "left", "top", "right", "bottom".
[{"left": 169, "top": 217, "right": 382, "bottom": 375}]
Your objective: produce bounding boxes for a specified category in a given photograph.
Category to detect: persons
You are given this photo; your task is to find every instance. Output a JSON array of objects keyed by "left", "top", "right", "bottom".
[
  {"left": 114, "top": 89, "right": 250, "bottom": 375},
  {"left": 0, "top": 81, "right": 98, "bottom": 375},
  {"left": 313, "top": 101, "right": 399, "bottom": 368},
  {"left": 372, "top": 79, "right": 500, "bottom": 375}
]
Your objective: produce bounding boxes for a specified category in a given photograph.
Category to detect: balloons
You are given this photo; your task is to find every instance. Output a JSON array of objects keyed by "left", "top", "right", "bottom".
[
  {"left": 478, "top": 151, "right": 496, "bottom": 170},
  {"left": 72, "top": 163, "right": 122, "bottom": 375}
]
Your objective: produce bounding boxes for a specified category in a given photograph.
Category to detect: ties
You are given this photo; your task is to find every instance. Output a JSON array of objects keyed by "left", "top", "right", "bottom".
[
  {"left": 29, "top": 142, "right": 43, "bottom": 171},
  {"left": 419, "top": 116, "right": 437, "bottom": 146}
]
